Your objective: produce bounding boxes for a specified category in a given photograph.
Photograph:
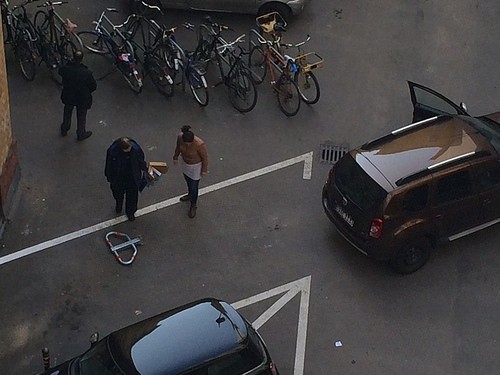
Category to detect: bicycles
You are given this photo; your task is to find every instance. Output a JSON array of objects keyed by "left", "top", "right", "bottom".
[{"left": 0, "top": 0, "right": 326, "bottom": 117}]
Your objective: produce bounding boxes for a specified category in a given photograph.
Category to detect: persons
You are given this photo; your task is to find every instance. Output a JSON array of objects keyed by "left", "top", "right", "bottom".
[
  {"left": 58, "top": 50, "right": 97, "bottom": 141},
  {"left": 104, "top": 137, "right": 148, "bottom": 222},
  {"left": 173, "top": 125, "right": 208, "bottom": 218}
]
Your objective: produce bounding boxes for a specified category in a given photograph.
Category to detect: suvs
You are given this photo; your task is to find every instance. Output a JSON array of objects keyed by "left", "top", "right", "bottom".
[{"left": 322, "top": 80, "right": 500, "bottom": 275}]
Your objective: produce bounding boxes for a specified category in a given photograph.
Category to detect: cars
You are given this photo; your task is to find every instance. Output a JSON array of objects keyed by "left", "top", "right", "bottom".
[
  {"left": 37, "top": 296, "right": 282, "bottom": 375},
  {"left": 130, "top": 0, "right": 305, "bottom": 23}
]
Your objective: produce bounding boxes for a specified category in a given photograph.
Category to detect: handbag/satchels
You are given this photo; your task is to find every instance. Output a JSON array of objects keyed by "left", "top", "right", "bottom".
[
  {"left": 149, "top": 162, "right": 168, "bottom": 174},
  {"left": 152, "top": 167, "right": 162, "bottom": 180},
  {"left": 148, "top": 173, "right": 156, "bottom": 181},
  {"left": 139, "top": 176, "right": 147, "bottom": 193}
]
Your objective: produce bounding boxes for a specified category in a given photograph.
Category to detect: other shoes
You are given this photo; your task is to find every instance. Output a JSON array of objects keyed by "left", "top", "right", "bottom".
[
  {"left": 129, "top": 215, "right": 135, "bottom": 222},
  {"left": 116, "top": 207, "right": 122, "bottom": 213},
  {"left": 78, "top": 131, "right": 92, "bottom": 140},
  {"left": 61, "top": 130, "right": 66, "bottom": 136},
  {"left": 189, "top": 206, "right": 198, "bottom": 217},
  {"left": 180, "top": 195, "right": 189, "bottom": 201}
]
